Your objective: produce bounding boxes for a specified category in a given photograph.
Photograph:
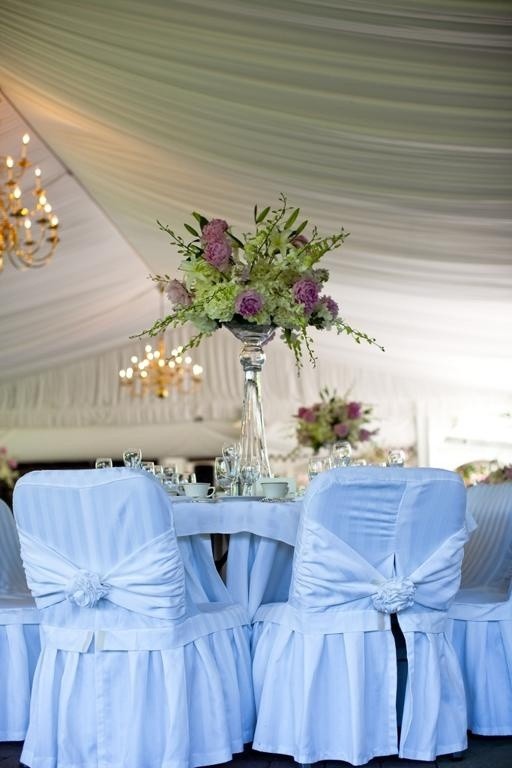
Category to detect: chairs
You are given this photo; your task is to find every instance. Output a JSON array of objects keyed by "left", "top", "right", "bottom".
[
  {"left": 0, "top": 497, "right": 42, "bottom": 742},
  {"left": 251, "top": 464, "right": 480, "bottom": 767},
  {"left": 445, "top": 482, "right": 512, "bottom": 737},
  {"left": 11, "top": 466, "right": 255, "bottom": 768}
]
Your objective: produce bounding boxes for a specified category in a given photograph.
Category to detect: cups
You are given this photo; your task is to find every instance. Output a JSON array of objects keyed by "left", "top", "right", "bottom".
[
  {"left": 181, "top": 482, "right": 216, "bottom": 501},
  {"left": 261, "top": 482, "right": 290, "bottom": 500}
]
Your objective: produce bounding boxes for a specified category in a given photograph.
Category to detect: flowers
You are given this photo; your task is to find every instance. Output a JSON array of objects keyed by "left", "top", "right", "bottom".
[
  {"left": 128, "top": 192, "right": 387, "bottom": 377},
  {"left": 291, "top": 381, "right": 382, "bottom": 455},
  {"left": 0, "top": 446, "right": 22, "bottom": 491}
]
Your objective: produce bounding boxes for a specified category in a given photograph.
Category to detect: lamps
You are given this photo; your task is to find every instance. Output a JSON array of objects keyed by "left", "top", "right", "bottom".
[
  {"left": 117, "top": 281, "right": 204, "bottom": 400},
  {"left": 0, "top": 133, "right": 60, "bottom": 276}
]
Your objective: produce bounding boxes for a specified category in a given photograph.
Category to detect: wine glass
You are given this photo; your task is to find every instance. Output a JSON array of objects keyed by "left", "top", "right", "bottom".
[{"left": 94, "top": 441, "right": 405, "bottom": 497}]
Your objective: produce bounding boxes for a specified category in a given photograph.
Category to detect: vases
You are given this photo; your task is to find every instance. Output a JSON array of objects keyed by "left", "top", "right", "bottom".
[{"left": 225, "top": 319, "right": 276, "bottom": 495}]
